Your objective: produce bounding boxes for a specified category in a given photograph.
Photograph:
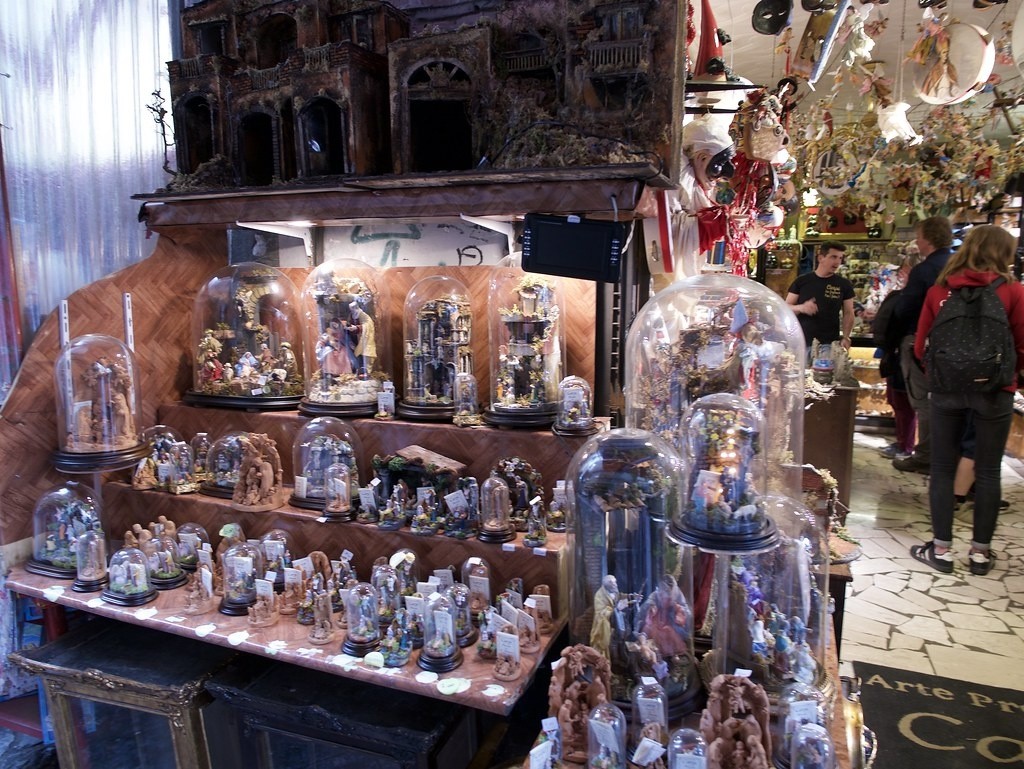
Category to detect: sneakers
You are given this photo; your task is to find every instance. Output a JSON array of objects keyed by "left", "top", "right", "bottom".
[
  {"left": 952, "top": 487, "right": 1010, "bottom": 529},
  {"left": 910, "top": 538, "right": 953, "bottom": 574},
  {"left": 968, "top": 546, "right": 998, "bottom": 576}
]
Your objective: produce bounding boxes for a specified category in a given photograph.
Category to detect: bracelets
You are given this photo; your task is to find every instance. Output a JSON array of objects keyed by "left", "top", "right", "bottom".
[{"left": 840, "top": 334, "right": 852, "bottom": 344}]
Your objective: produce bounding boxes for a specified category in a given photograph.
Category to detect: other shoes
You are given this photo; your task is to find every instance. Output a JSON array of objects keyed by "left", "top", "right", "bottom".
[
  {"left": 892, "top": 453, "right": 929, "bottom": 476},
  {"left": 878, "top": 440, "right": 911, "bottom": 459}
]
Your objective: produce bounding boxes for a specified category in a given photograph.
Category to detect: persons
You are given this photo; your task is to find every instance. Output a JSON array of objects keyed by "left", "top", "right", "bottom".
[
  {"left": 892, "top": 217, "right": 956, "bottom": 475},
  {"left": 549, "top": 574, "right": 840, "bottom": 769},
  {"left": 77, "top": 513, "right": 553, "bottom": 682},
  {"left": 74, "top": 354, "right": 131, "bottom": 443},
  {"left": 785, "top": 240, "right": 855, "bottom": 365},
  {"left": 145, "top": 426, "right": 571, "bottom": 547},
  {"left": 950, "top": 436, "right": 1008, "bottom": 527},
  {"left": 872, "top": 287, "right": 918, "bottom": 460},
  {"left": 195, "top": 260, "right": 592, "bottom": 434},
  {"left": 908, "top": 225, "right": 1024, "bottom": 576}
]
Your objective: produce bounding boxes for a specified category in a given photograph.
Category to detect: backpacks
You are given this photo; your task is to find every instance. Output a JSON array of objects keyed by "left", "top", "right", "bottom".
[{"left": 921, "top": 273, "right": 1018, "bottom": 397}]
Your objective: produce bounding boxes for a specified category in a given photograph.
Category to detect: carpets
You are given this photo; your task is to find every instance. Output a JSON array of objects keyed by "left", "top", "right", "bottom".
[{"left": 852, "top": 660, "right": 1023, "bottom": 769}]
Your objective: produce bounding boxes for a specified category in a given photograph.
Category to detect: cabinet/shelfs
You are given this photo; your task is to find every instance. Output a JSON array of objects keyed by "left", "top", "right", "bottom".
[
  {"left": 6, "top": 618, "right": 241, "bottom": 769},
  {"left": 4, "top": 401, "right": 612, "bottom": 717},
  {"left": 206, "top": 660, "right": 478, "bottom": 768}
]
